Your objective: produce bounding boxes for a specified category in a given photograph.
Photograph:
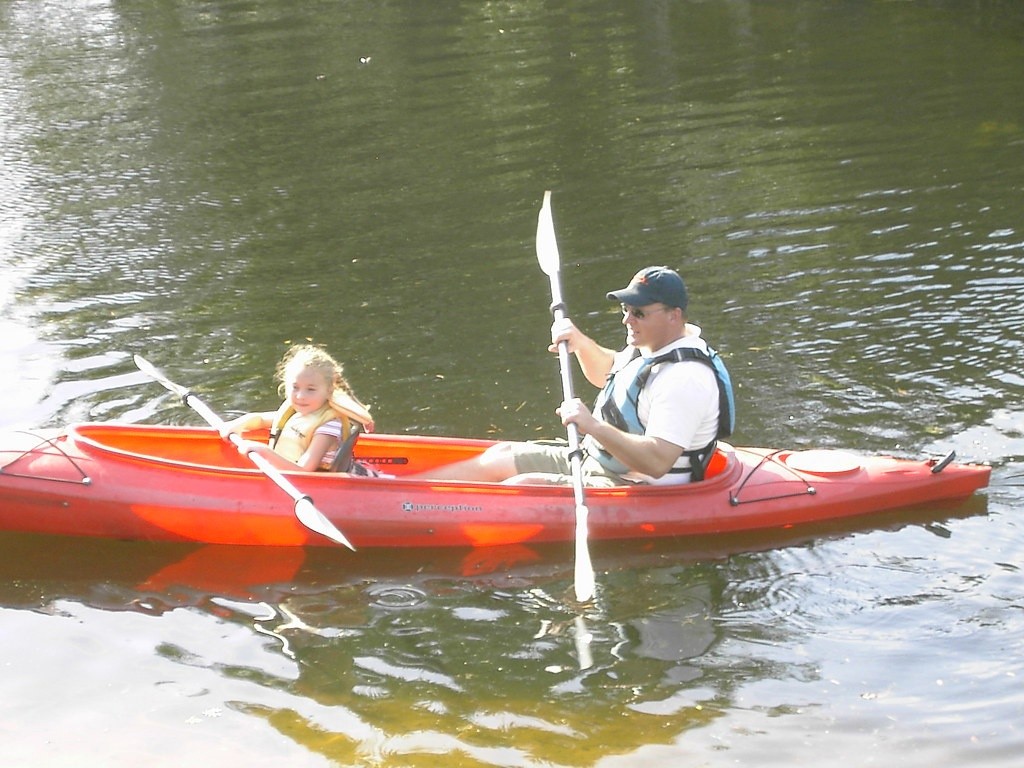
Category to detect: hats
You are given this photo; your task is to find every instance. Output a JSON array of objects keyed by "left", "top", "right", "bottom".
[{"left": 606, "top": 265, "right": 688, "bottom": 312}]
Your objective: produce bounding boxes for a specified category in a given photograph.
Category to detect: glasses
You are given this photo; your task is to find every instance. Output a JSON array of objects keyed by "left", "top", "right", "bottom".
[{"left": 620, "top": 302, "right": 673, "bottom": 319}]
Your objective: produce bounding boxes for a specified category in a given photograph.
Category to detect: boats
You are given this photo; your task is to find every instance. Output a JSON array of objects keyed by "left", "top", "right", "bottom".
[{"left": 1, "top": 417, "right": 990, "bottom": 552}]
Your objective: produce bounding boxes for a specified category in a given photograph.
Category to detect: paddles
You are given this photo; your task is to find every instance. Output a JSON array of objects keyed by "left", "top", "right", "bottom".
[
  {"left": 535, "top": 187, "right": 598, "bottom": 614},
  {"left": 134, "top": 355, "right": 354, "bottom": 555}
]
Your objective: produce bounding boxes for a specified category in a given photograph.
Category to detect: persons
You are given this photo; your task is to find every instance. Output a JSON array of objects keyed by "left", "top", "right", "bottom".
[
  {"left": 350, "top": 266, "right": 734, "bottom": 485},
  {"left": 220, "top": 342, "right": 351, "bottom": 479}
]
